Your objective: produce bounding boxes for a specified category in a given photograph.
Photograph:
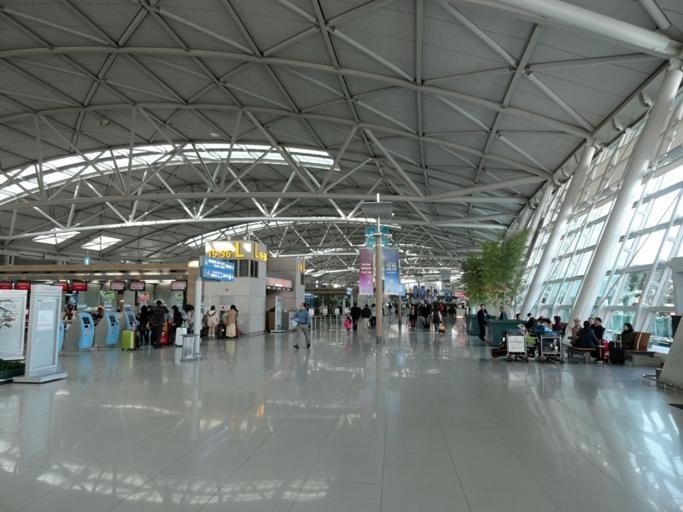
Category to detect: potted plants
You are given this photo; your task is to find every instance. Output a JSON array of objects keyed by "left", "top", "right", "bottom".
[
  {"left": 475, "top": 226, "right": 533, "bottom": 347},
  {"left": 448, "top": 249, "right": 496, "bottom": 335}
]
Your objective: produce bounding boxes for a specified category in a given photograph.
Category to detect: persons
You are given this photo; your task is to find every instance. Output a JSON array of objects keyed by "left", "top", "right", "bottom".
[
  {"left": 290, "top": 303, "right": 311, "bottom": 350},
  {"left": 369, "top": 303, "right": 377, "bottom": 329},
  {"left": 476, "top": 304, "right": 490, "bottom": 342},
  {"left": 344, "top": 316, "right": 351, "bottom": 332},
  {"left": 499, "top": 306, "right": 508, "bottom": 320},
  {"left": 349, "top": 302, "right": 360, "bottom": 331},
  {"left": 359, "top": 304, "right": 371, "bottom": 330},
  {"left": 380, "top": 289, "right": 464, "bottom": 334},
  {"left": 514, "top": 312, "right": 634, "bottom": 366},
  {"left": 62, "top": 294, "right": 240, "bottom": 350}
]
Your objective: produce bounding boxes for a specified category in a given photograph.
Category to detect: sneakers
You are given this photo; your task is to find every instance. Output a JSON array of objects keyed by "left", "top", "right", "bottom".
[
  {"left": 293, "top": 344, "right": 298, "bottom": 349},
  {"left": 307, "top": 344, "right": 309, "bottom": 348}
]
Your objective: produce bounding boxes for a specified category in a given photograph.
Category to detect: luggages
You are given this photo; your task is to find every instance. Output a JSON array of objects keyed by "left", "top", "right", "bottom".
[
  {"left": 175, "top": 319, "right": 187, "bottom": 346},
  {"left": 217, "top": 324, "right": 226, "bottom": 339},
  {"left": 612, "top": 334, "right": 624, "bottom": 365},
  {"left": 121, "top": 331, "right": 134, "bottom": 351}
]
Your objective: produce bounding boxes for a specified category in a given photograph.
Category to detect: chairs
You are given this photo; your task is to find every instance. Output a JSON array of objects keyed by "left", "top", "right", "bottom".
[{"left": 504, "top": 324, "right": 652, "bottom": 364}]
